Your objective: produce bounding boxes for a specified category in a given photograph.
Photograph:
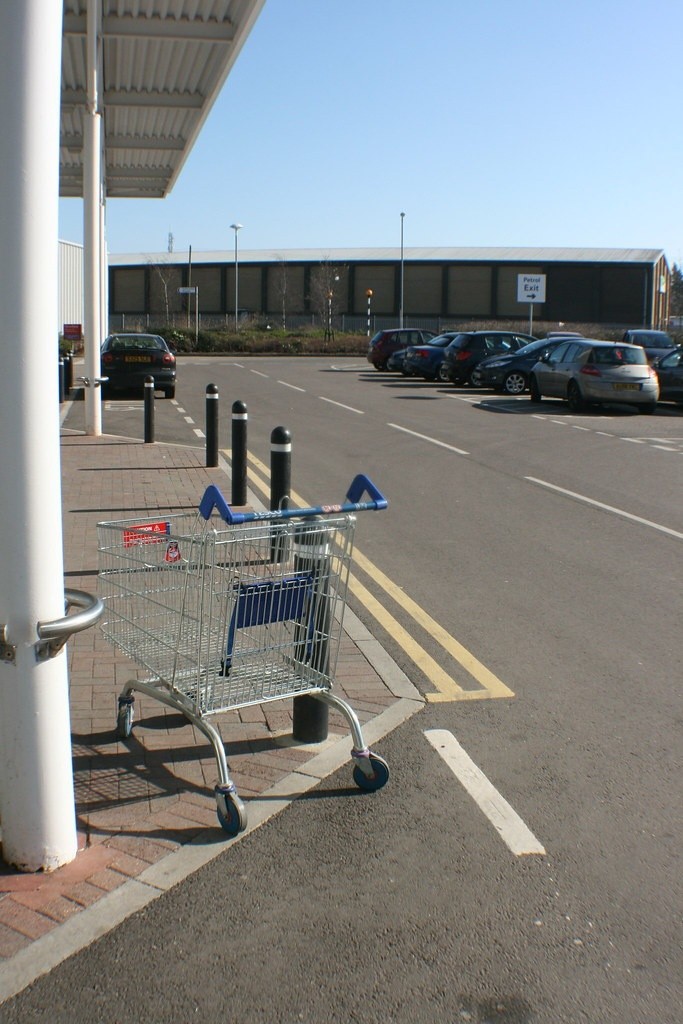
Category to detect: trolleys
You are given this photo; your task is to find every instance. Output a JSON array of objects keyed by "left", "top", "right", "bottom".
[{"left": 98, "top": 473, "right": 389, "bottom": 836}]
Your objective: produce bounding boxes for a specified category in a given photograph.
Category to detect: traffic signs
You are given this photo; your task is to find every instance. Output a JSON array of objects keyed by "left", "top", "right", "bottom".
[{"left": 177, "top": 287, "right": 196, "bottom": 294}]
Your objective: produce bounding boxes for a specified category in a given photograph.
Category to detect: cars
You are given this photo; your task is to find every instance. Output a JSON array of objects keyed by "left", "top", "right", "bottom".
[
  {"left": 625, "top": 329, "right": 675, "bottom": 365},
  {"left": 650, "top": 346, "right": 682, "bottom": 404},
  {"left": 402, "top": 332, "right": 459, "bottom": 382},
  {"left": 528, "top": 340, "right": 660, "bottom": 414},
  {"left": 471, "top": 332, "right": 592, "bottom": 395},
  {"left": 385, "top": 334, "right": 443, "bottom": 376},
  {"left": 367, "top": 327, "right": 438, "bottom": 371},
  {"left": 443, "top": 330, "right": 538, "bottom": 388},
  {"left": 101, "top": 334, "right": 176, "bottom": 400}
]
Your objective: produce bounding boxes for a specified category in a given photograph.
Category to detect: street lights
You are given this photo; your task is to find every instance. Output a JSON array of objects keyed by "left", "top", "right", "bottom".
[
  {"left": 399, "top": 212, "right": 406, "bottom": 329},
  {"left": 230, "top": 224, "right": 242, "bottom": 332}
]
